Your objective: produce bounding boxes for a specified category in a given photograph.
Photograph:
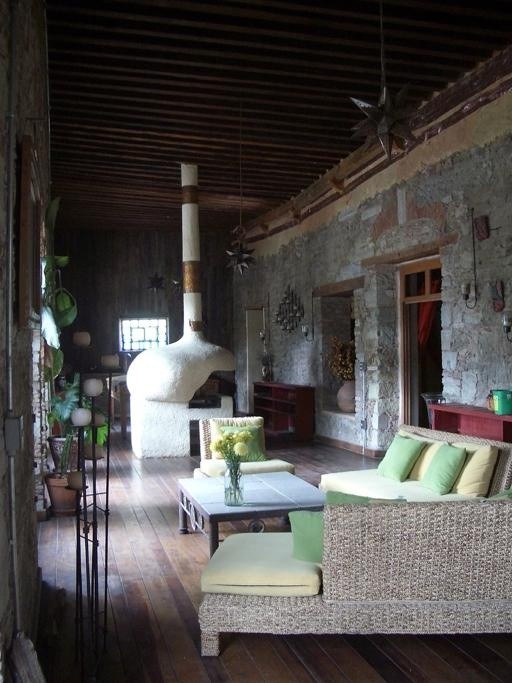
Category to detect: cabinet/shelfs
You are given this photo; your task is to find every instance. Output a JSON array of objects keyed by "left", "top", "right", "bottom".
[
  {"left": 254, "top": 383, "right": 314, "bottom": 441},
  {"left": 427, "top": 404, "right": 512, "bottom": 442}
]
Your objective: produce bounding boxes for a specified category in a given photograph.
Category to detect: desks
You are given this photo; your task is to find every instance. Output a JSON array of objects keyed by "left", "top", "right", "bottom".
[{"left": 105, "top": 375, "right": 220, "bottom": 439}]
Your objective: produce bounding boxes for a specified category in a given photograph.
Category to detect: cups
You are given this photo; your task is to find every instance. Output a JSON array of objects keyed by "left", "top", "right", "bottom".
[
  {"left": 492, "top": 391, "right": 512, "bottom": 416},
  {"left": 487, "top": 390, "right": 494, "bottom": 411}
]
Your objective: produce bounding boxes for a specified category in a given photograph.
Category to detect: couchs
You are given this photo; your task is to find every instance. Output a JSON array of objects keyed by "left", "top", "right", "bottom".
[{"left": 319, "top": 423, "right": 512, "bottom": 505}]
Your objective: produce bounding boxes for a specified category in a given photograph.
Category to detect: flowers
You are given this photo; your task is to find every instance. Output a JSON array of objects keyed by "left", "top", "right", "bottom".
[
  {"left": 209, "top": 430, "right": 254, "bottom": 506},
  {"left": 319, "top": 335, "right": 355, "bottom": 381}
]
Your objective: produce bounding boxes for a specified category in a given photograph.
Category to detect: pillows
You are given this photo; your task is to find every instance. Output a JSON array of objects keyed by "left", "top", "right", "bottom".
[
  {"left": 210, "top": 416, "right": 267, "bottom": 462},
  {"left": 288, "top": 510, "right": 324, "bottom": 563},
  {"left": 326, "top": 490, "right": 407, "bottom": 505},
  {"left": 377, "top": 430, "right": 499, "bottom": 498}
]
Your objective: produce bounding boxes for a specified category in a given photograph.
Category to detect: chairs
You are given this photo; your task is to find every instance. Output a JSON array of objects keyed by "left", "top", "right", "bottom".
[{"left": 194, "top": 416, "right": 295, "bottom": 478}]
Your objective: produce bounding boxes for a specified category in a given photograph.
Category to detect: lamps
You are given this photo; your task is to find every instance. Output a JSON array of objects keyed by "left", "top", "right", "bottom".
[
  {"left": 302, "top": 291, "right": 314, "bottom": 342},
  {"left": 501, "top": 310, "right": 512, "bottom": 343},
  {"left": 461, "top": 207, "right": 477, "bottom": 310},
  {"left": 259, "top": 291, "right": 270, "bottom": 346}
]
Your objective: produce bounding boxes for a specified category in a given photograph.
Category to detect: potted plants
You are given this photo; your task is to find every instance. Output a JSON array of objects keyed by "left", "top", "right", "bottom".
[{"left": 45, "top": 388, "right": 108, "bottom": 513}]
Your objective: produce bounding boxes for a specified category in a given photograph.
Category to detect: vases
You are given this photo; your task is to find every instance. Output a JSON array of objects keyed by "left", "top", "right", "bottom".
[
  {"left": 336, "top": 381, "right": 354, "bottom": 413},
  {"left": 223, "top": 463, "right": 244, "bottom": 506}
]
